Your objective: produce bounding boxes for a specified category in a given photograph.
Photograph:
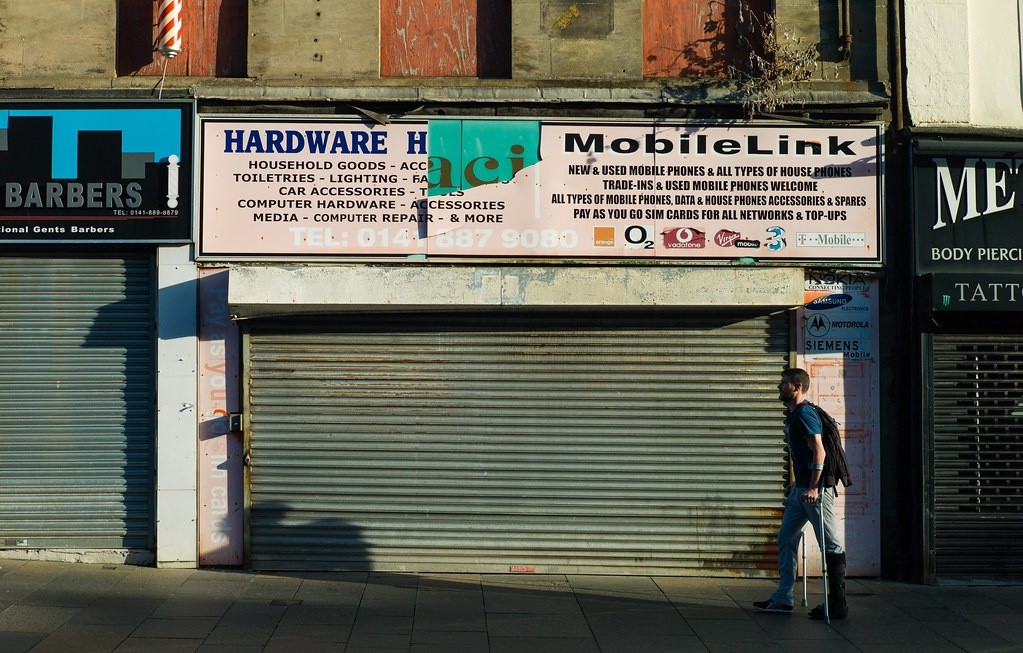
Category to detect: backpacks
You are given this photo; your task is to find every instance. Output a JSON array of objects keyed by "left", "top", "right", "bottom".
[{"left": 790, "top": 401, "right": 852, "bottom": 497}]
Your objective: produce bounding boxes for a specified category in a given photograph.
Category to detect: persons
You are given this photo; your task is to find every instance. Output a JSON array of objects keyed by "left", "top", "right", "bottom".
[{"left": 753, "top": 368, "right": 848, "bottom": 621}]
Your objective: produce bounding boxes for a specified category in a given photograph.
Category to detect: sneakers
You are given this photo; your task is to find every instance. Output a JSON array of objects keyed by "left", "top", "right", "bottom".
[{"left": 753, "top": 599, "right": 794, "bottom": 613}]
